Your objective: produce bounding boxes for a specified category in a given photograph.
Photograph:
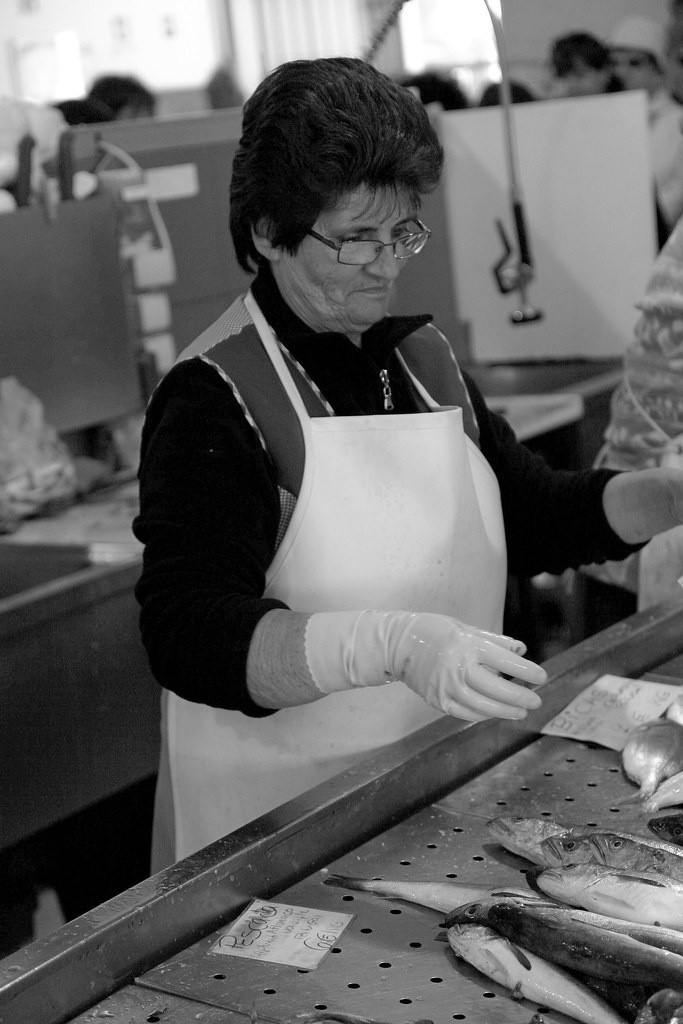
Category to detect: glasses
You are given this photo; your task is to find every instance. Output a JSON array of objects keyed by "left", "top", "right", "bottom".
[{"left": 308, "top": 217, "right": 432, "bottom": 265}]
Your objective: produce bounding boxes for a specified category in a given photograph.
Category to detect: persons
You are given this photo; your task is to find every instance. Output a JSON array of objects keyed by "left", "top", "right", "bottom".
[
  {"left": 406, "top": 32, "right": 683, "bottom": 251},
  {"left": 130, "top": 56, "right": 683, "bottom": 878},
  {"left": 571, "top": 212, "right": 683, "bottom": 639},
  {"left": 47, "top": 75, "right": 153, "bottom": 124}
]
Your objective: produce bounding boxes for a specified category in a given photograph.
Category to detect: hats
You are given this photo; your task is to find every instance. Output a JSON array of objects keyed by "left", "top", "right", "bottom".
[{"left": 604, "top": 13, "right": 669, "bottom": 62}]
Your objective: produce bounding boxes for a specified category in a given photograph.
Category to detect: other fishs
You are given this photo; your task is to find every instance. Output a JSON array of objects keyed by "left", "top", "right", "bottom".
[{"left": 325, "top": 719, "right": 683, "bottom": 1024}]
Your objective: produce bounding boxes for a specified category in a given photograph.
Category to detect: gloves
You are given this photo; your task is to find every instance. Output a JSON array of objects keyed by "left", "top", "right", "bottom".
[{"left": 303, "top": 609, "right": 547, "bottom": 722}]
